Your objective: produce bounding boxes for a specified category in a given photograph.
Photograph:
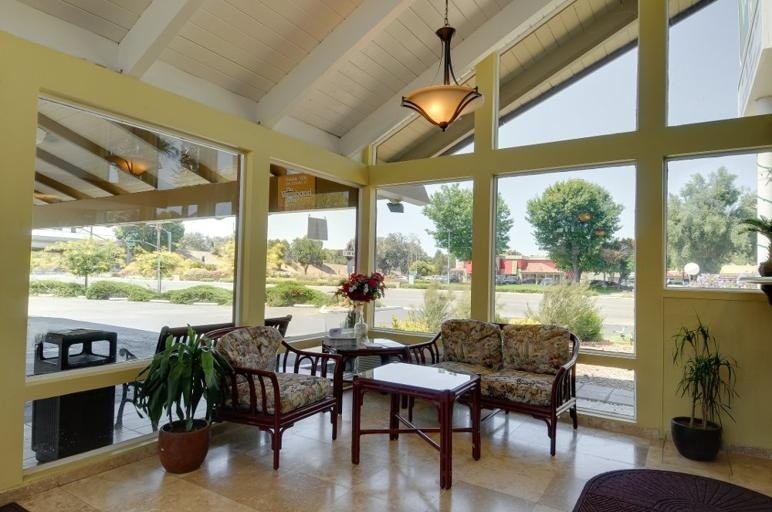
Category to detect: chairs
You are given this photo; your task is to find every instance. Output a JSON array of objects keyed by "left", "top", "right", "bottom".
[{"left": 203, "top": 325, "right": 344, "bottom": 472}]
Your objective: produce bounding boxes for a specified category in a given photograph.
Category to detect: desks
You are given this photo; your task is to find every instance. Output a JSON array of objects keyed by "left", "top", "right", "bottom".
[
  {"left": 352, "top": 362, "right": 482, "bottom": 489},
  {"left": 320, "top": 336, "right": 410, "bottom": 426}
]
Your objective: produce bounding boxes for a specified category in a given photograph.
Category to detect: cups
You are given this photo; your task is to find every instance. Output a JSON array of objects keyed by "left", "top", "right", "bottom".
[{"left": 339, "top": 320, "right": 348, "bottom": 329}]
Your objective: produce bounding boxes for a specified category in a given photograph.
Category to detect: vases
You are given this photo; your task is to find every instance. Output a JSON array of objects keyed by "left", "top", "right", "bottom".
[{"left": 352, "top": 301, "right": 372, "bottom": 347}]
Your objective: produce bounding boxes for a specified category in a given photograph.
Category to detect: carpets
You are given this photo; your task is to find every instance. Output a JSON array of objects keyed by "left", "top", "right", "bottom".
[{"left": 570, "top": 468, "right": 772, "bottom": 512}]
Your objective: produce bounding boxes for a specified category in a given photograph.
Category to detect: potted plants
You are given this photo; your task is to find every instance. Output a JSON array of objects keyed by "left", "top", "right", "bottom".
[
  {"left": 133, "top": 323, "right": 234, "bottom": 474},
  {"left": 669, "top": 303, "right": 744, "bottom": 461}
]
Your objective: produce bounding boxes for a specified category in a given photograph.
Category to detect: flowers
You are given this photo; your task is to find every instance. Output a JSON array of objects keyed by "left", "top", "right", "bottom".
[{"left": 335, "top": 272, "right": 385, "bottom": 304}]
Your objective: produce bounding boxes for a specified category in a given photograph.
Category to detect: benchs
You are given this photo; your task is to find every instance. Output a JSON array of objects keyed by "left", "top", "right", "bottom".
[
  {"left": 115, "top": 315, "right": 295, "bottom": 432},
  {"left": 406, "top": 319, "right": 582, "bottom": 457}
]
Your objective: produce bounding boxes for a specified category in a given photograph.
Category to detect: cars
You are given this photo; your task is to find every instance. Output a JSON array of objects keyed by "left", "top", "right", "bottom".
[
  {"left": 666, "top": 279, "right": 689, "bottom": 288},
  {"left": 391, "top": 273, "right": 559, "bottom": 286}
]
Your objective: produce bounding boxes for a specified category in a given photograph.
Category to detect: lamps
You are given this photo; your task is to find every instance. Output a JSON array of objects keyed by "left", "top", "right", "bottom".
[
  {"left": 401, "top": 0, "right": 485, "bottom": 134},
  {"left": 109, "top": 122, "right": 162, "bottom": 176}
]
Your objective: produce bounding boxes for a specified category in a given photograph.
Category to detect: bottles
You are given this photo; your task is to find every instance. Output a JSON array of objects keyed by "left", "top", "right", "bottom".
[{"left": 353, "top": 312, "right": 369, "bottom": 347}]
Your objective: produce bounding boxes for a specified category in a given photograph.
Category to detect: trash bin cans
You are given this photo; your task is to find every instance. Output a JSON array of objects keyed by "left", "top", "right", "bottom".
[{"left": 31, "top": 329, "right": 116, "bottom": 467}]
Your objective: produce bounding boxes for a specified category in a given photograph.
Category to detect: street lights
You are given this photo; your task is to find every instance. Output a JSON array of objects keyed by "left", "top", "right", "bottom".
[{"left": 433, "top": 220, "right": 451, "bottom": 285}]
